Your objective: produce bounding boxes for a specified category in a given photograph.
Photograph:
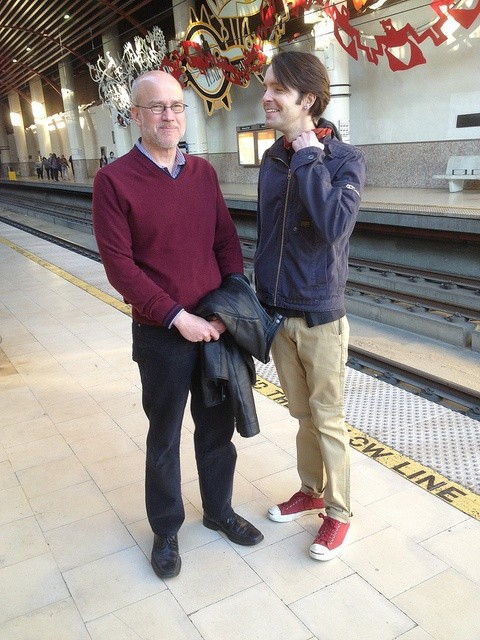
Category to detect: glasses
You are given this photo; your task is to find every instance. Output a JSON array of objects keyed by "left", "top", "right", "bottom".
[{"left": 134, "top": 102, "right": 187, "bottom": 112}]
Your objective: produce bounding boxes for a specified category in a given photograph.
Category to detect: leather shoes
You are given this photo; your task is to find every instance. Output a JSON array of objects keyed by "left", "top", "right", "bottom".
[
  {"left": 151, "top": 532, "right": 182, "bottom": 578},
  {"left": 204, "top": 505, "right": 265, "bottom": 546}
]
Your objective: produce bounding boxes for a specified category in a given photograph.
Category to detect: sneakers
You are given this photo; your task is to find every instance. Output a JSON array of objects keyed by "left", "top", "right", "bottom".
[
  {"left": 266, "top": 490, "right": 327, "bottom": 522},
  {"left": 309, "top": 513, "right": 352, "bottom": 561}
]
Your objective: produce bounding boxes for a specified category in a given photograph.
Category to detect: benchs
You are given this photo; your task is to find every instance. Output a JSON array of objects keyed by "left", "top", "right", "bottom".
[{"left": 432, "top": 155, "right": 480, "bottom": 192}]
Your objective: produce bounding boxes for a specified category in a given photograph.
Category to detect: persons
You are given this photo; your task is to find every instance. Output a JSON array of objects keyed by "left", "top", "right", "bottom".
[
  {"left": 35, "top": 151, "right": 119, "bottom": 182},
  {"left": 251, "top": 51, "right": 369, "bottom": 562},
  {"left": 91, "top": 69, "right": 265, "bottom": 580}
]
